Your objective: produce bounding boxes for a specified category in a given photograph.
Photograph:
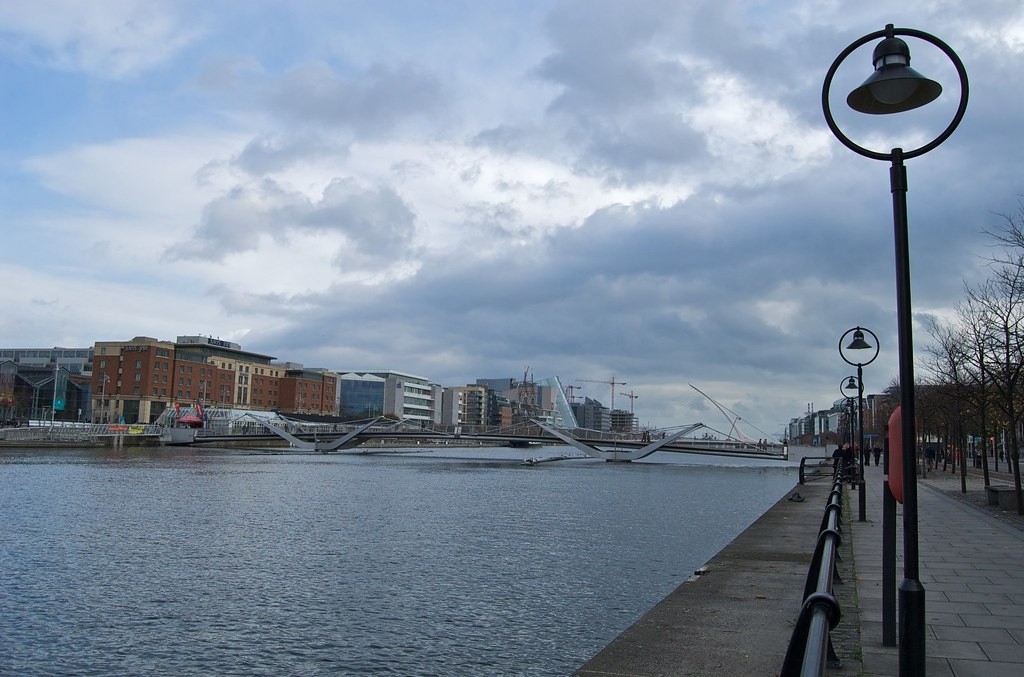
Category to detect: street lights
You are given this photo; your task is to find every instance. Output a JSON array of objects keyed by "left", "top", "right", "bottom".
[
  {"left": 838, "top": 326, "right": 880, "bottom": 520},
  {"left": 840, "top": 375, "right": 865, "bottom": 489},
  {"left": 821, "top": 23, "right": 973, "bottom": 677},
  {"left": 840, "top": 397, "right": 858, "bottom": 464}
]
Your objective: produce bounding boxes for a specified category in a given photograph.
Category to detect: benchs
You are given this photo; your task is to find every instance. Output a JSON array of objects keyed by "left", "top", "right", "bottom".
[{"left": 983, "top": 485, "right": 1019, "bottom": 510}]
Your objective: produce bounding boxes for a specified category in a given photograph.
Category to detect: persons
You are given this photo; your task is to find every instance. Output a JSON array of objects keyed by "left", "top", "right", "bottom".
[
  {"left": 756, "top": 437, "right": 788, "bottom": 453},
  {"left": 641, "top": 430, "right": 665, "bottom": 443},
  {"left": 833, "top": 441, "right": 1004, "bottom": 473}
]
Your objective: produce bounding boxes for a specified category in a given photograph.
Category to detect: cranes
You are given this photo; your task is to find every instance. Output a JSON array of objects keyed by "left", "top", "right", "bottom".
[
  {"left": 511, "top": 364, "right": 542, "bottom": 408},
  {"left": 573, "top": 376, "right": 627, "bottom": 409},
  {"left": 619, "top": 390, "right": 639, "bottom": 412},
  {"left": 563, "top": 385, "right": 584, "bottom": 403}
]
